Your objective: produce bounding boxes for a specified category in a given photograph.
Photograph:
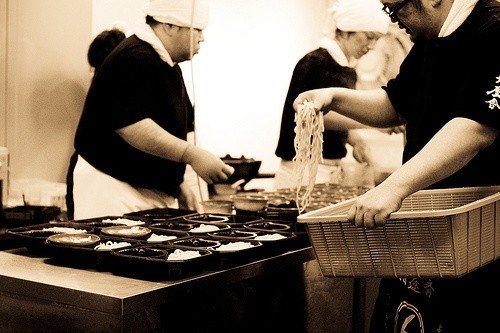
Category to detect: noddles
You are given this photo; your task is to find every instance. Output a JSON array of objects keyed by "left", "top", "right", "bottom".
[{"left": 292, "top": 100, "right": 323, "bottom": 216}]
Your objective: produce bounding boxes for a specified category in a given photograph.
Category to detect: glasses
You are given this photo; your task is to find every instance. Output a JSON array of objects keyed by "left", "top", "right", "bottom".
[{"left": 381, "top": 1, "right": 408, "bottom": 18}]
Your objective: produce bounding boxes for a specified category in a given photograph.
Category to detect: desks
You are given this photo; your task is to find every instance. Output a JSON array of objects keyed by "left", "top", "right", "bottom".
[{"left": 0, "top": 234, "right": 368, "bottom": 333}]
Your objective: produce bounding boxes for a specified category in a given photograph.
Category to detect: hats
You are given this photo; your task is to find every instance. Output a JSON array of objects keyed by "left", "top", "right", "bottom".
[
  {"left": 144, "top": 1, "right": 207, "bottom": 31},
  {"left": 331, "top": 0, "right": 389, "bottom": 34}
]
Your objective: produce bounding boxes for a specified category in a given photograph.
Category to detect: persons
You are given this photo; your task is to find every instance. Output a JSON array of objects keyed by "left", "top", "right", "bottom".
[
  {"left": 66, "top": 0, "right": 234, "bottom": 221},
  {"left": 294, "top": 0, "right": 500, "bottom": 333},
  {"left": 276, "top": 0, "right": 391, "bottom": 190}
]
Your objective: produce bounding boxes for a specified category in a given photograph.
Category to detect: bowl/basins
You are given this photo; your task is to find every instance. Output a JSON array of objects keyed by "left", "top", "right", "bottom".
[
  {"left": 268, "top": 208, "right": 306, "bottom": 221},
  {"left": 173, "top": 239, "right": 221, "bottom": 249},
  {"left": 182, "top": 224, "right": 231, "bottom": 236},
  {"left": 183, "top": 215, "right": 229, "bottom": 223},
  {"left": 117, "top": 248, "right": 168, "bottom": 258},
  {"left": 214, "top": 184, "right": 239, "bottom": 195},
  {"left": 248, "top": 222, "right": 291, "bottom": 231},
  {"left": 101, "top": 226, "right": 152, "bottom": 240},
  {"left": 246, "top": 232, "right": 298, "bottom": 242},
  {"left": 212, "top": 158, "right": 262, "bottom": 186},
  {"left": 91, "top": 240, "right": 137, "bottom": 251},
  {"left": 207, "top": 240, "right": 263, "bottom": 252},
  {"left": 137, "top": 233, "right": 184, "bottom": 243},
  {"left": 47, "top": 234, "right": 101, "bottom": 248},
  {"left": 201, "top": 200, "right": 233, "bottom": 215},
  {"left": 234, "top": 207, "right": 268, "bottom": 220},
  {"left": 148, "top": 222, "right": 194, "bottom": 231},
  {"left": 230, "top": 197, "right": 269, "bottom": 211},
  {"left": 158, "top": 248, "right": 212, "bottom": 261},
  {"left": 207, "top": 230, "right": 257, "bottom": 241}
]
